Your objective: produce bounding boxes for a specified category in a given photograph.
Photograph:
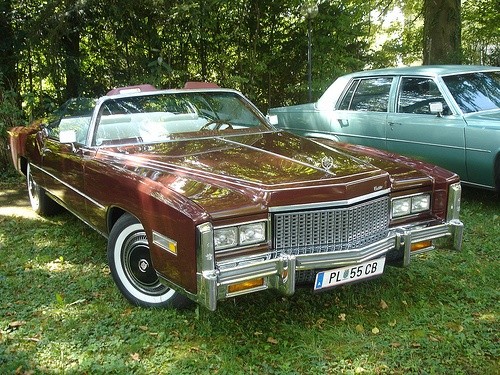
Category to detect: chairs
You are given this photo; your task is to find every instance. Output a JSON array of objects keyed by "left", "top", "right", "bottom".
[
  {"left": 97, "top": 115, "right": 140, "bottom": 139},
  {"left": 159, "top": 113, "right": 209, "bottom": 133}
]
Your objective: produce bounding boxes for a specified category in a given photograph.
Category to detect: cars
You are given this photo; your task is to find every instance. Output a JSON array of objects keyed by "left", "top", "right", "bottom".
[
  {"left": 267, "top": 63, "right": 500, "bottom": 205},
  {"left": 11, "top": 81, "right": 465, "bottom": 313}
]
